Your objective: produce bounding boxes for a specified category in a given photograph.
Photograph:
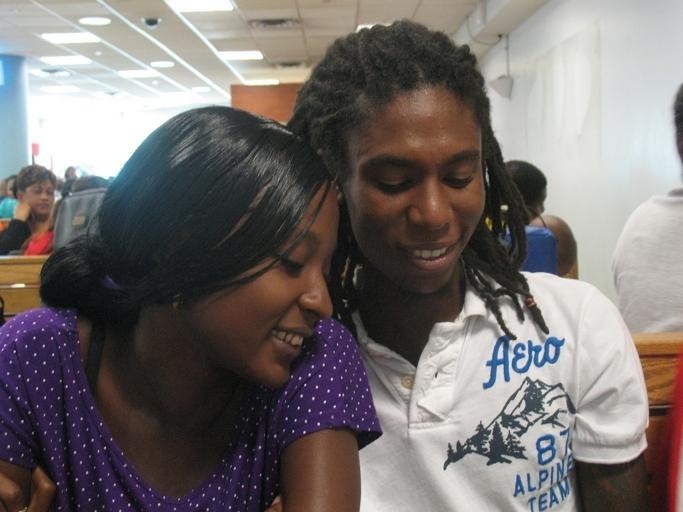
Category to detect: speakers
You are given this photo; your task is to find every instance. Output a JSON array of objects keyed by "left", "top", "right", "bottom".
[{"left": 487, "top": 75, "right": 514, "bottom": 99}]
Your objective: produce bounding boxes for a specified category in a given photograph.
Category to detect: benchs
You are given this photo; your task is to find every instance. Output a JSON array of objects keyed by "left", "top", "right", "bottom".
[
  {"left": 628, "top": 329, "right": 679, "bottom": 512},
  {"left": 1, "top": 181, "right": 82, "bottom": 331}
]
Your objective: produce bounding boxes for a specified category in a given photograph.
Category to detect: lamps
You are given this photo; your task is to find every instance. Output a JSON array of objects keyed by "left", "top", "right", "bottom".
[{"left": 488, "top": 73, "right": 516, "bottom": 100}]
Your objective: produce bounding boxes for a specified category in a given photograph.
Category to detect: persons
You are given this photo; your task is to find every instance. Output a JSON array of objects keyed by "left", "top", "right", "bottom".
[
  {"left": 611, "top": 83, "right": 683, "bottom": 336},
  {"left": 0, "top": 164, "right": 113, "bottom": 255},
  {"left": 502, "top": 159, "right": 548, "bottom": 224},
  {"left": 0, "top": 105, "right": 384, "bottom": 512},
  {"left": 288, "top": 19, "right": 654, "bottom": 512}
]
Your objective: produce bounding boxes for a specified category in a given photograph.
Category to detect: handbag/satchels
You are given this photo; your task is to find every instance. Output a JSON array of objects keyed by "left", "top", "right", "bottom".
[{"left": 53, "top": 188, "right": 108, "bottom": 254}]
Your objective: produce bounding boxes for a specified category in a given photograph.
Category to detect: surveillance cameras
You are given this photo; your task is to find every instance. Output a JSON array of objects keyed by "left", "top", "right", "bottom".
[{"left": 140, "top": 16, "right": 162, "bottom": 30}]
